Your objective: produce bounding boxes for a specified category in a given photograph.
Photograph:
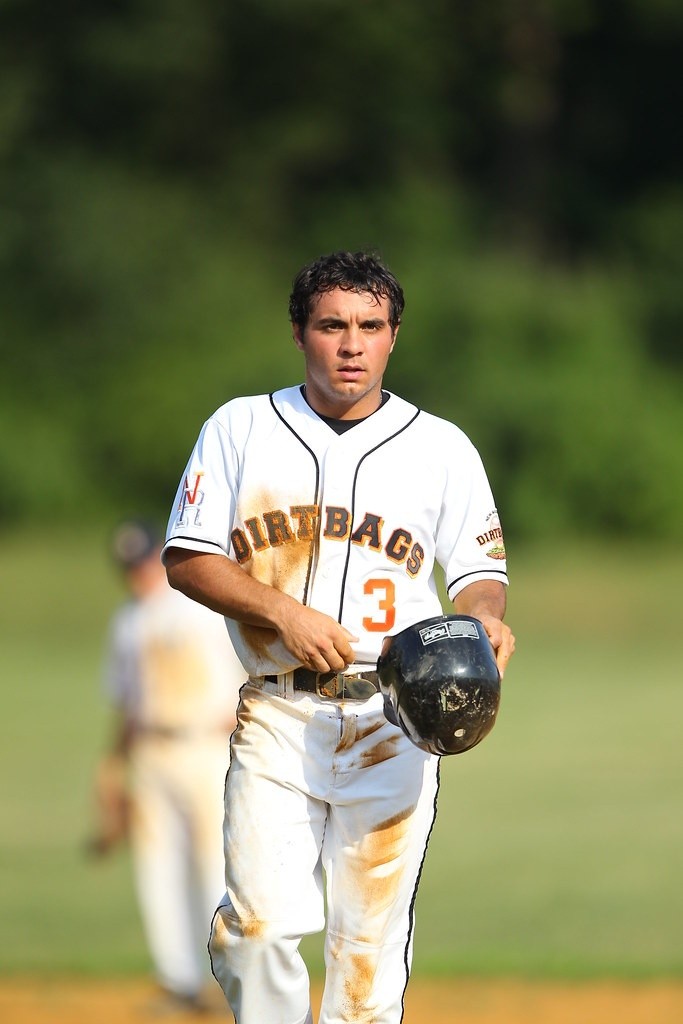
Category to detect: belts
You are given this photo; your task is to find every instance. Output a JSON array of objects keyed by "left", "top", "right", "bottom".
[{"left": 266, "top": 667, "right": 380, "bottom": 699}]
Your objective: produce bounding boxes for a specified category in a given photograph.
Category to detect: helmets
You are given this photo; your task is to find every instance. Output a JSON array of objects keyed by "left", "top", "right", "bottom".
[{"left": 376, "top": 614, "right": 501, "bottom": 757}]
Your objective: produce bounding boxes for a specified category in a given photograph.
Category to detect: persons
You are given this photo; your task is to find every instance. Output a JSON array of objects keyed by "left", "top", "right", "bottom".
[
  {"left": 94, "top": 512, "right": 252, "bottom": 1018},
  {"left": 162, "top": 246, "right": 519, "bottom": 1024}
]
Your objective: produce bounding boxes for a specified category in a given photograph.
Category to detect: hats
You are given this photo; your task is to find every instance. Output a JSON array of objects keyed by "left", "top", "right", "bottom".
[{"left": 111, "top": 519, "right": 159, "bottom": 566}]
